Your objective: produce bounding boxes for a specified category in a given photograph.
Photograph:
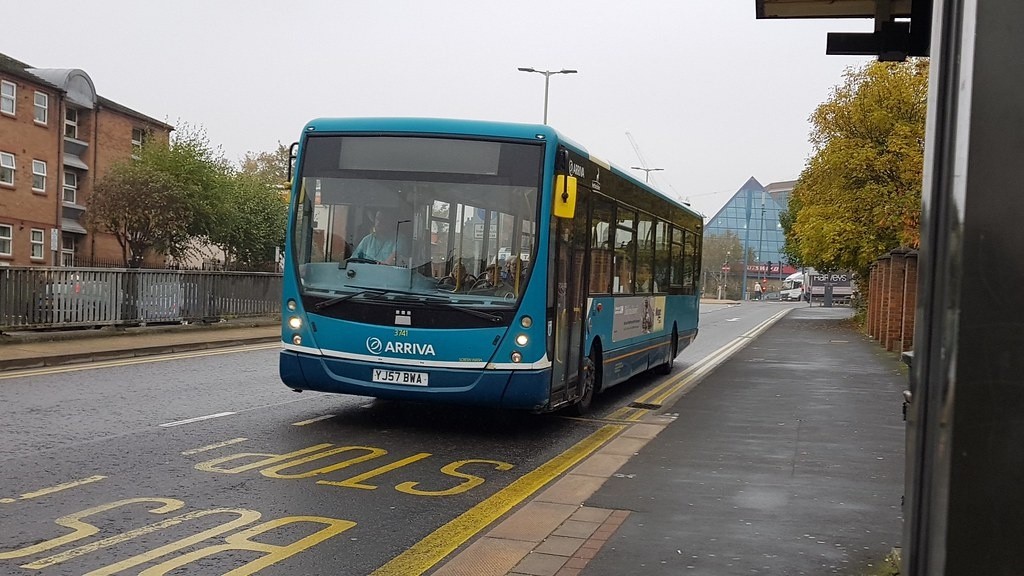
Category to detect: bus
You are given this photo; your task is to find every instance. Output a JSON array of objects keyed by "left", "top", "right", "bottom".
[{"left": 271, "top": 115, "right": 705, "bottom": 418}]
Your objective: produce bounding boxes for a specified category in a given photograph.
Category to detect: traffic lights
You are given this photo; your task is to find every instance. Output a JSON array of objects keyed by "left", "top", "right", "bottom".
[
  {"left": 767, "top": 261, "right": 773, "bottom": 275},
  {"left": 779, "top": 261, "right": 784, "bottom": 274}
]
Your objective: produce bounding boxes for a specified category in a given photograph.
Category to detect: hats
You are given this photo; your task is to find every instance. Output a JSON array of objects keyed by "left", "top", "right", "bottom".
[{"left": 486, "top": 264, "right": 502, "bottom": 271}]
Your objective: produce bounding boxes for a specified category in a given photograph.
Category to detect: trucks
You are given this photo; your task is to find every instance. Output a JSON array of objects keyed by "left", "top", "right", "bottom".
[{"left": 780, "top": 266, "right": 859, "bottom": 302}]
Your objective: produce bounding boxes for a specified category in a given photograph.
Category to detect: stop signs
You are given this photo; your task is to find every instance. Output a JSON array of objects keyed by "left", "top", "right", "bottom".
[{"left": 722, "top": 264, "right": 731, "bottom": 273}]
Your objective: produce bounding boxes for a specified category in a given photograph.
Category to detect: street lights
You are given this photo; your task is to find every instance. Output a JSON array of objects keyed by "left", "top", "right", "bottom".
[
  {"left": 517, "top": 67, "right": 578, "bottom": 124},
  {"left": 631, "top": 166, "right": 664, "bottom": 183}
]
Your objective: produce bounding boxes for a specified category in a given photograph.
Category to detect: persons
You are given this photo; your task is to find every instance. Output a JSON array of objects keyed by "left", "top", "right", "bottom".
[
  {"left": 753, "top": 282, "right": 763, "bottom": 300},
  {"left": 349, "top": 207, "right": 408, "bottom": 266},
  {"left": 444, "top": 262, "right": 469, "bottom": 291},
  {"left": 504, "top": 256, "right": 524, "bottom": 288},
  {"left": 636, "top": 263, "right": 659, "bottom": 293},
  {"left": 476, "top": 264, "right": 513, "bottom": 293}
]
